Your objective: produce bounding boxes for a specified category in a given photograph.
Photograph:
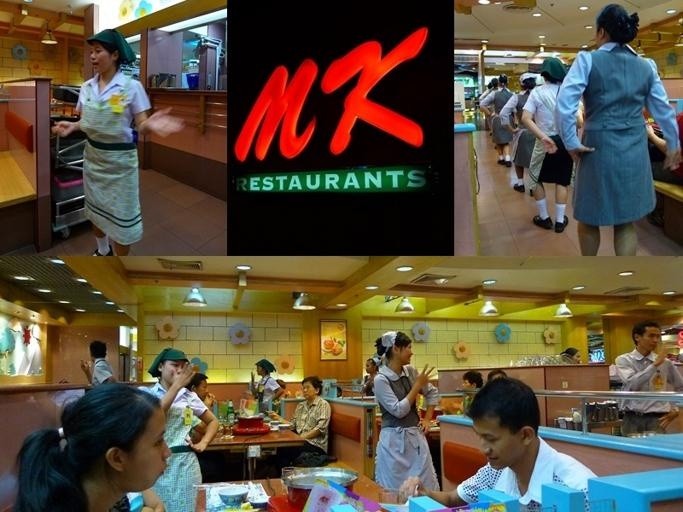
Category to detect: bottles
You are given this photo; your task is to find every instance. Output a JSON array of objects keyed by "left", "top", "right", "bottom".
[
  {"left": 150, "top": 72, "right": 176, "bottom": 87},
  {"left": 255, "top": 397, "right": 259, "bottom": 414},
  {"left": 226, "top": 399, "right": 235, "bottom": 422},
  {"left": 572, "top": 409, "right": 582, "bottom": 423},
  {"left": 591, "top": 406, "right": 619, "bottom": 421}
]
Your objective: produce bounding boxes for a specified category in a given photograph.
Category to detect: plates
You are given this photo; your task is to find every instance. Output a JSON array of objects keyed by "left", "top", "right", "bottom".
[
  {"left": 281, "top": 468, "right": 359, "bottom": 491},
  {"left": 270, "top": 421, "right": 291, "bottom": 428},
  {"left": 321, "top": 338, "right": 337, "bottom": 352},
  {"left": 162, "top": 86, "right": 188, "bottom": 90}
]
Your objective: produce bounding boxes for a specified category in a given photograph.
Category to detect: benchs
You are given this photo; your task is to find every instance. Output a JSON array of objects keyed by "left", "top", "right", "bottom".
[
  {"left": 1, "top": 77, "right": 50, "bottom": 255},
  {"left": 190, "top": 393, "right": 683, "bottom": 511}
]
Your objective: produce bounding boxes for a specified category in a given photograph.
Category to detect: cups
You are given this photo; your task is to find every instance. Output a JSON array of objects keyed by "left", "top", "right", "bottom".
[
  {"left": 222, "top": 425, "right": 230, "bottom": 438},
  {"left": 280, "top": 467, "right": 296, "bottom": 486},
  {"left": 296, "top": 392, "right": 301, "bottom": 399},
  {"left": 378, "top": 489, "right": 408, "bottom": 505}
]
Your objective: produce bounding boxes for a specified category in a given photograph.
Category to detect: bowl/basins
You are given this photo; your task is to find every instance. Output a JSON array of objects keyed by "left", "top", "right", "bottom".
[
  {"left": 285, "top": 487, "right": 353, "bottom": 509},
  {"left": 248, "top": 495, "right": 267, "bottom": 510},
  {"left": 218, "top": 486, "right": 248, "bottom": 508},
  {"left": 186, "top": 73, "right": 198, "bottom": 90}
]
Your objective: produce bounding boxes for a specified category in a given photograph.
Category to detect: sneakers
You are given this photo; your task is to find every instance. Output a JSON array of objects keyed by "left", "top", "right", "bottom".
[
  {"left": 496, "top": 159, "right": 513, "bottom": 168},
  {"left": 532, "top": 214, "right": 553, "bottom": 229},
  {"left": 513, "top": 183, "right": 525, "bottom": 192},
  {"left": 554, "top": 215, "right": 569, "bottom": 232}
]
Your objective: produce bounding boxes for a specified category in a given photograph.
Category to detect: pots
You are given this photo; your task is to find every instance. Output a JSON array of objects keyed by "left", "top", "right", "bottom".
[{"left": 237, "top": 417, "right": 263, "bottom": 428}]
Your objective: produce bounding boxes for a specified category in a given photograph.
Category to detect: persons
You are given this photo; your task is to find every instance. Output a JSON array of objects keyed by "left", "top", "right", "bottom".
[
  {"left": 137, "top": 348, "right": 218, "bottom": 512},
  {"left": 645, "top": 112, "right": 683, "bottom": 226},
  {"left": 80, "top": 341, "right": 116, "bottom": 383},
  {"left": 270, "top": 376, "right": 331, "bottom": 478},
  {"left": 251, "top": 360, "right": 284, "bottom": 412},
  {"left": 457, "top": 371, "right": 483, "bottom": 415},
  {"left": 14, "top": 383, "right": 172, "bottom": 512},
  {"left": 52, "top": 30, "right": 186, "bottom": 256},
  {"left": 126, "top": 489, "right": 165, "bottom": 512},
  {"left": 374, "top": 331, "right": 441, "bottom": 492},
  {"left": 488, "top": 369, "right": 507, "bottom": 381},
  {"left": 615, "top": 321, "right": 683, "bottom": 437},
  {"left": 560, "top": 348, "right": 580, "bottom": 364},
  {"left": 185, "top": 373, "right": 215, "bottom": 474},
  {"left": 401, "top": 377, "right": 599, "bottom": 512},
  {"left": 554, "top": 4, "right": 683, "bottom": 256},
  {"left": 478, "top": 58, "right": 583, "bottom": 232},
  {"left": 360, "top": 357, "right": 382, "bottom": 396}
]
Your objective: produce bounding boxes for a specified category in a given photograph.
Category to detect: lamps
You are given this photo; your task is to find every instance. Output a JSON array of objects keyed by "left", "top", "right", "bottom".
[
  {"left": 552, "top": 302, "right": 571, "bottom": 318},
  {"left": 290, "top": 292, "right": 316, "bottom": 312},
  {"left": 385, "top": 295, "right": 415, "bottom": 313},
  {"left": 461, "top": 297, "right": 502, "bottom": 319},
  {"left": 182, "top": 286, "right": 207, "bottom": 310},
  {"left": 40, "top": 22, "right": 60, "bottom": 46}
]
